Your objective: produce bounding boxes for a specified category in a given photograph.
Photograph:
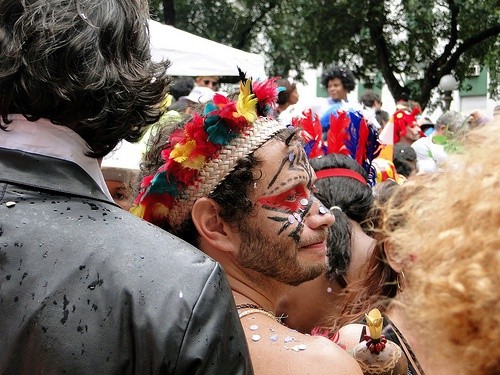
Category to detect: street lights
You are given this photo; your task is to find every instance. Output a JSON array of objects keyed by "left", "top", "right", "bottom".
[{"left": 440, "top": 74, "right": 457, "bottom": 111}]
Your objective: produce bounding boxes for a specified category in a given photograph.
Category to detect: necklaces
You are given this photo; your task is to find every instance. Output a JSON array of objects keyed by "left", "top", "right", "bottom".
[{"left": 237, "top": 302, "right": 288, "bottom": 323}]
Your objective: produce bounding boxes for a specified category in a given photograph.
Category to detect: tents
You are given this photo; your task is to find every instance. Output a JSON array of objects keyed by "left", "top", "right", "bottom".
[{"left": 146, "top": 18, "right": 268, "bottom": 82}]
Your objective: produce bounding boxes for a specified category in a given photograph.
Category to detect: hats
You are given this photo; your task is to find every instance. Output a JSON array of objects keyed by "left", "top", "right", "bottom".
[{"left": 180, "top": 87, "right": 228, "bottom": 104}]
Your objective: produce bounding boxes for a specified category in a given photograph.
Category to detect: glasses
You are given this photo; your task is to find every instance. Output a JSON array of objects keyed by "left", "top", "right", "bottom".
[{"left": 203, "top": 79, "right": 218, "bottom": 87}]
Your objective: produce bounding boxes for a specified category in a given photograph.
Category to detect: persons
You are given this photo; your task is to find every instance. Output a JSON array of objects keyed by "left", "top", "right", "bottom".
[
  {"left": 319, "top": 109, "right": 500, "bottom": 375},
  {"left": 161, "top": 76, "right": 221, "bottom": 115},
  {"left": 319, "top": 66, "right": 500, "bottom": 236},
  {"left": 120, "top": 65, "right": 364, "bottom": 375},
  {"left": 274, "top": 77, "right": 300, "bottom": 121},
  {"left": 274, "top": 109, "right": 387, "bottom": 334},
  {"left": 0, "top": 0, "right": 255, "bottom": 375}
]
